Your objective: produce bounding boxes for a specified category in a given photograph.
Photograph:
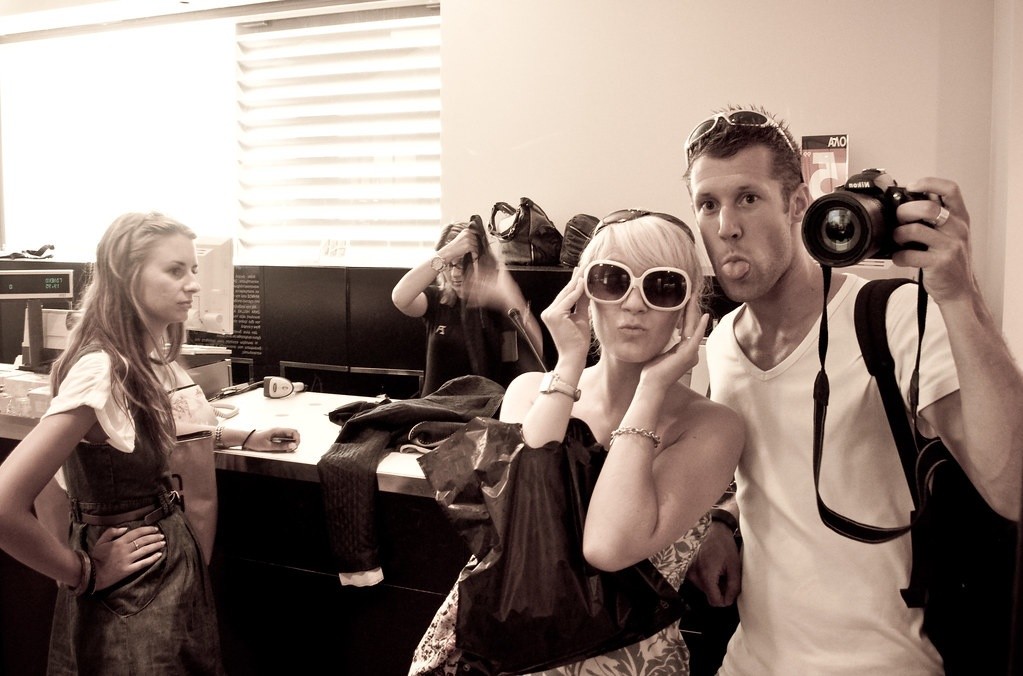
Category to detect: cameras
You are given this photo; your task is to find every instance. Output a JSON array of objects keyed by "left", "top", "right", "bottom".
[{"left": 801, "top": 167, "right": 932, "bottom": 267}]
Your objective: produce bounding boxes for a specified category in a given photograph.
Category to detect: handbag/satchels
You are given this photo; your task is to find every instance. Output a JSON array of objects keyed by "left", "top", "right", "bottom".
[
  {"left": 455, "top": 425, "right": 686, "bottom": 676},
  {"left": 559, "top": 214, "right": 600, "bottom": 267},
  {"left": 856, "top": 273, "right": 1023, "bottom": 676},
  {"left": 487, "top": 197, "right": 563, "bottom": 265},
  {"left": 34, "top": 430, "right": 217, "bottom": 587}
]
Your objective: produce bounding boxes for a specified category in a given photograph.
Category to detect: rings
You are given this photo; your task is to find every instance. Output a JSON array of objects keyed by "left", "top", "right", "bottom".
[
  {"left": 682, "top": 337, "right": 692, "bottom": 341},
  {"left": 930, "top": 204, "right": 950, "bottom": 227},
  {"left": 133, "top": 541, "right": 140, "bottom": 549}
]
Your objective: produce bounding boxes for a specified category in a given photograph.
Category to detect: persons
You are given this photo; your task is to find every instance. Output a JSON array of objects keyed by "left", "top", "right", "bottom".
[
  {"left": 408, "top": 210, "right": 747, "bottom": 676},
  {"left": 391, "top": 223, "right": 543, "bottom": 399},
  {"left": 682, "top": 105, "right": 1023, "bottom": 676},
  {"left": 0, "top": 211, "right": 301, "bottom": 676}
]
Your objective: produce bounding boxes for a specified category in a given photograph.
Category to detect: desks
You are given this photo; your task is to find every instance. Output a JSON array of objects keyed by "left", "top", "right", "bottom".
[{"left": 200, "top": 381, "right": 466, "bottom": 676}]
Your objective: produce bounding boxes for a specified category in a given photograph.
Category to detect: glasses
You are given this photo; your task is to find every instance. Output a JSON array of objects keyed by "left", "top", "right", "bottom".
[
  {"left": 444, "top": 261, "right": 463, "bottom": 272},
  {"left": 582, "top": 260, "right": 693, "bottom": 312},
  {"left": 684, "top": 110, "right": 796, "bottom": 171},
  {"left": 589, "top": 208, "right": 695, "bottom": 246}
]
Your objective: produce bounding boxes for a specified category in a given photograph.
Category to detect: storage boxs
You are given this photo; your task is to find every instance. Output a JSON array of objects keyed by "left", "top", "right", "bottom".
[{"left": 160, "top": 344, "right": 234, "bottom": 403}]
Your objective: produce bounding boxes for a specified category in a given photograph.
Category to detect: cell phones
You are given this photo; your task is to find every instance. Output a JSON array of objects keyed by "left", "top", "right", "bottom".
[{"left": 271, "top": 437, "right": 296, "bottom": 443}]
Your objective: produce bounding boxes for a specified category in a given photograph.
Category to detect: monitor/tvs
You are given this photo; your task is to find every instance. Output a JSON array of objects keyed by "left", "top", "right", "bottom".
[{"left": 180, "top": 236, "right": 234, "bottom": 335}]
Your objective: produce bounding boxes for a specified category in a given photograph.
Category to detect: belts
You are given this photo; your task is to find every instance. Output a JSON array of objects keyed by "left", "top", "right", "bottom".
[{"left": 69, "top": 489, "right": 181, "bottom": 526}]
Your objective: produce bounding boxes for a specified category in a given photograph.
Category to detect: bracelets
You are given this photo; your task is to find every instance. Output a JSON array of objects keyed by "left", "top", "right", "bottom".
[
  {"left": 215, "top": 426, "right": 229, "bottom": 449},
  {"left": 243, "top": 429, "right": 256, "bottom": 446},
  {"left": 67, "top": 550, "right": 97, "bottom": 596},
  {"left": 707, "top": 506, "right": 743, "bottom": 554},
  {"left": 609, "top": 425, "right": 661, "bottom": 448}
]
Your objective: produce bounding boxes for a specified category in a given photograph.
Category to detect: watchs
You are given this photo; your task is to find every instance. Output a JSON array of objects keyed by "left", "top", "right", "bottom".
[
  {"left": 430, "top": 252, "right": 447, "bottom": 272},
  {"left": 539, "top": 369, "right": 581, "bottom": 402}
]
